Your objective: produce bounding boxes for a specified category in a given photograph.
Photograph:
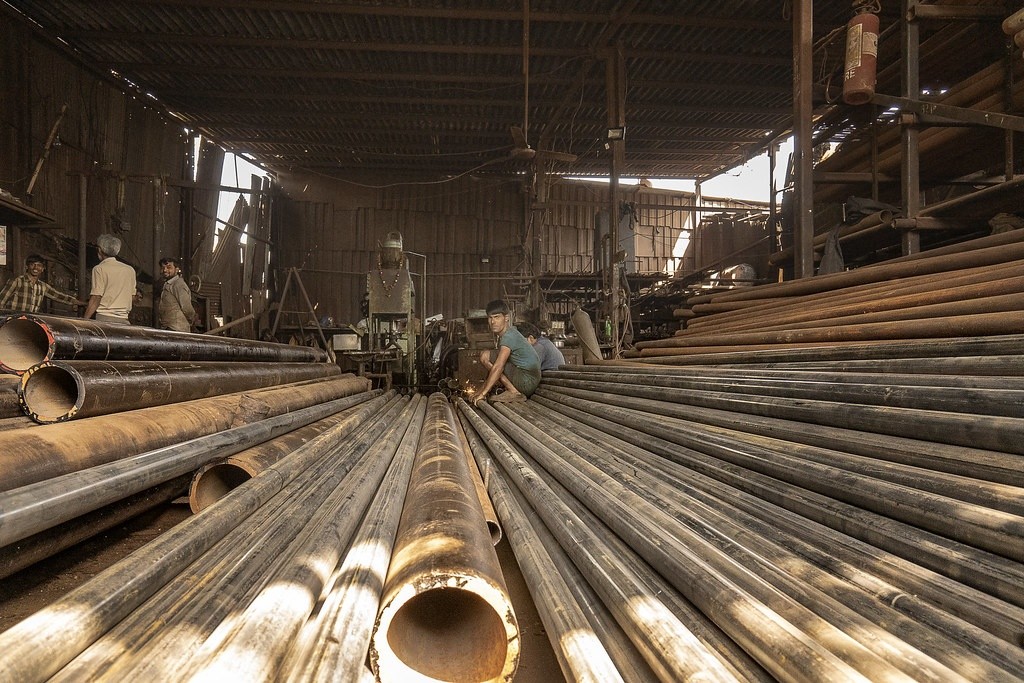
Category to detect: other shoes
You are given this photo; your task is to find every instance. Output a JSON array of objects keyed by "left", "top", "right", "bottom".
[{"left": 490, "top": 390, "right": 527, "bottom": 402}]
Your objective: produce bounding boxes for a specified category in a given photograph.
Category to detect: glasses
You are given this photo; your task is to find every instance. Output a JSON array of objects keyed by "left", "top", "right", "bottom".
[{"left": 95, "top": 244, "right": 100, "bottom": 249}]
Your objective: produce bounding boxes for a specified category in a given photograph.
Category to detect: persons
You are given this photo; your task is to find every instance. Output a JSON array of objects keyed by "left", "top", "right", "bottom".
[
  {"left": 158, "top": 257, "right": 197, "bottom": 332},
  {"left": 0, "top": 254, "right": 88, "bottom": 312},
  {"left": 84, "top": 234, "right": 137, "bottom": 324},
  {"left": 517, "top": 322, "right": 566, "bottom": 369},
  {"left": 469, "top": 299, "right": 542, "bottom": 407}
]
